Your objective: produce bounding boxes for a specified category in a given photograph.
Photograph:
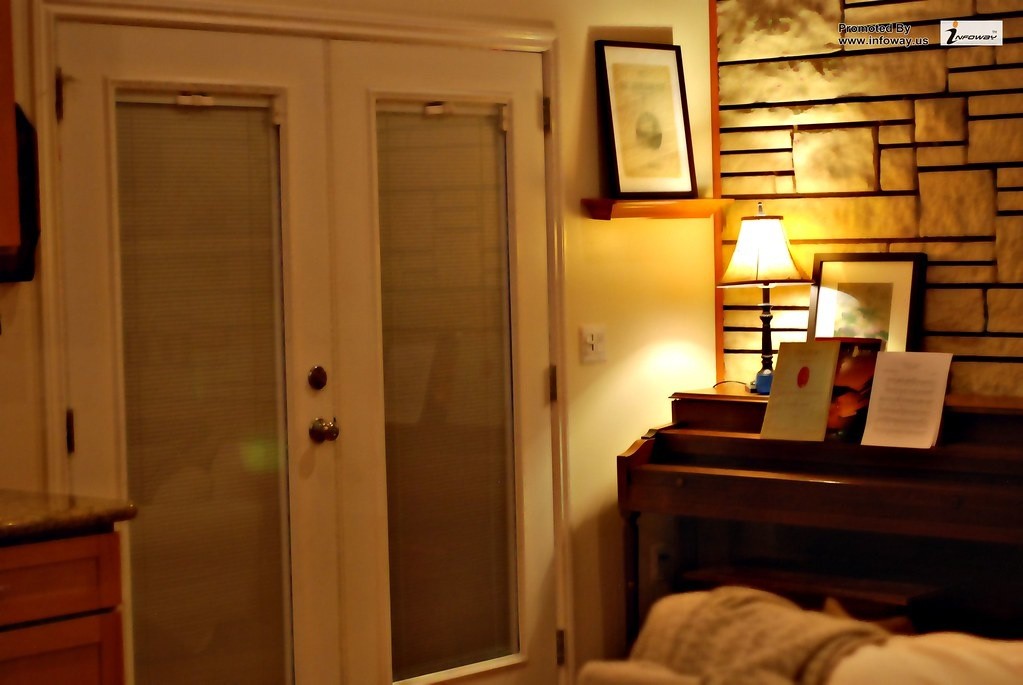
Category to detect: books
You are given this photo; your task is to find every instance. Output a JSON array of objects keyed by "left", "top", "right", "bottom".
[
  {"left": 808, "top": 336, "right": 888, "bottom": 445},
  {"left": 758, "top": 339, "right": 830, "bottom": 443}
]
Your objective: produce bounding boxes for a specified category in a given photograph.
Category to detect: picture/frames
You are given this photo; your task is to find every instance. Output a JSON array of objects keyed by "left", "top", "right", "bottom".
[
  {"left": 595, "top": 39, "right": 700, "bottom": 199},
  {"left": 807, "top": 251, "right": 929, "bottom": 363}
]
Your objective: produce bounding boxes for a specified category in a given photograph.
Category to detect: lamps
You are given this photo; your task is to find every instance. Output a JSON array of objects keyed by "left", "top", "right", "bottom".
[{"left": 716, "top": 197, "right": 814, "bottom": 393}]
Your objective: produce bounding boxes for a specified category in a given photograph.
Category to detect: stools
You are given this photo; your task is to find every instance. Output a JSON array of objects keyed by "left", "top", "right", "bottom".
[{"left": 684, "top": 555, "right": 939, "bottom": 635}]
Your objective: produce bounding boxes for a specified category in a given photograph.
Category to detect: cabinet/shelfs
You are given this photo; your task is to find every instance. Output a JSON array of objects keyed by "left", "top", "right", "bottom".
[{"left": 1, "top": 536, "right": 130, "bottom": 685}]
